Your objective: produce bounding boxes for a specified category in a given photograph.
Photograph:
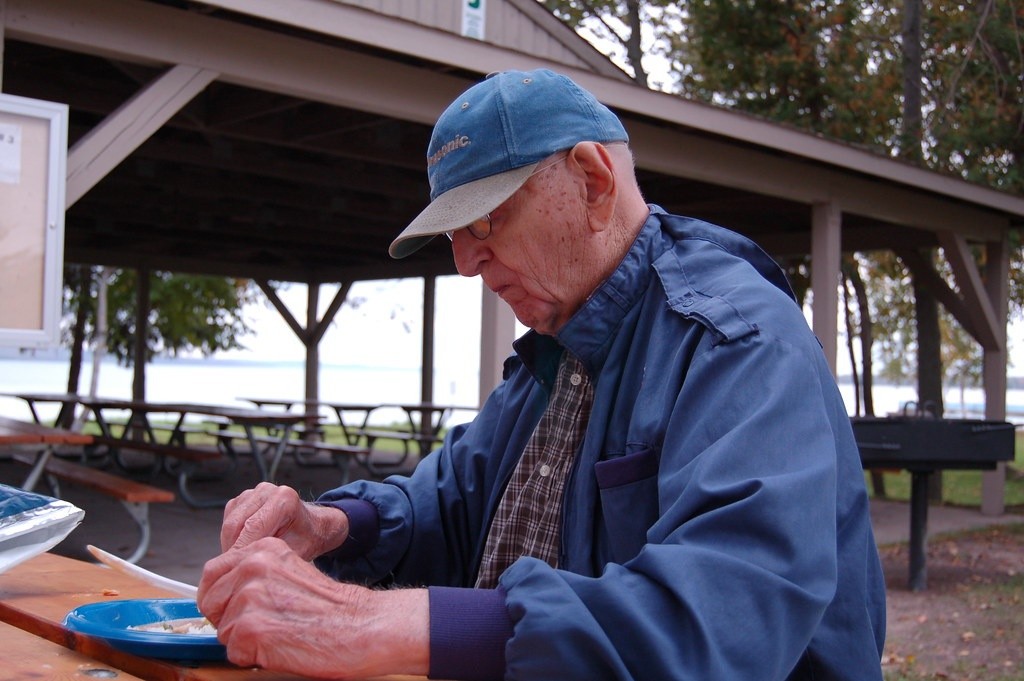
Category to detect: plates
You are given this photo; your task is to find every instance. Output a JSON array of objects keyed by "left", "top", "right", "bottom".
[{"left": 62, "top": 598, "right": 227, "bottom": 661}]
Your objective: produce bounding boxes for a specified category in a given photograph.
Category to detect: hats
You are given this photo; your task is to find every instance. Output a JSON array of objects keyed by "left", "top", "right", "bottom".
[{"left": 389, "top": 68, "right": 630, "bottom": 259}]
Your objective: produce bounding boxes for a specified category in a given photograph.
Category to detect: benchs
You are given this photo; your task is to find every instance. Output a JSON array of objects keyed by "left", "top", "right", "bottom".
[
  {"left": 348, "top": 429, "right": 442, "bottom": 444},
  {"left": 199, "top": 419, "right": 232, "bottom": 426},
  {"left": 203, "top": 431, "right": 367, "bottom": 456},
  {"left": 87, "top": 418, "right": 202, "bottom": 437},
  {"left": 16, "top": 454, "right": 173, "bottom": 503}
]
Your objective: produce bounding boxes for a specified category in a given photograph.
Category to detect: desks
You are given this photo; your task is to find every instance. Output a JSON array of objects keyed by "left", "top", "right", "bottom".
[{"left": 0, "top": 394, "right": 479, "bottom": 681}]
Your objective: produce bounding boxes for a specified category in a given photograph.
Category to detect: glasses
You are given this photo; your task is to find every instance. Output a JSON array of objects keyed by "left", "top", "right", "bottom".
[{"left": 444, "top": 155, "right": 568, "bottom": 242}]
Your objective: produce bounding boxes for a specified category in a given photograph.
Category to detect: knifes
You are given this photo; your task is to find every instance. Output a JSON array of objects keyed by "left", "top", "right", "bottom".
[{"left": 86, "top": 544, "right": 198, "bottom": 599}]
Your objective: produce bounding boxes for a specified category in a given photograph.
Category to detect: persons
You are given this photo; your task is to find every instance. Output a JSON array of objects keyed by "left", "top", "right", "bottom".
[{"left": 197, "top": 74, "right": 888, "bottom": 681}]
